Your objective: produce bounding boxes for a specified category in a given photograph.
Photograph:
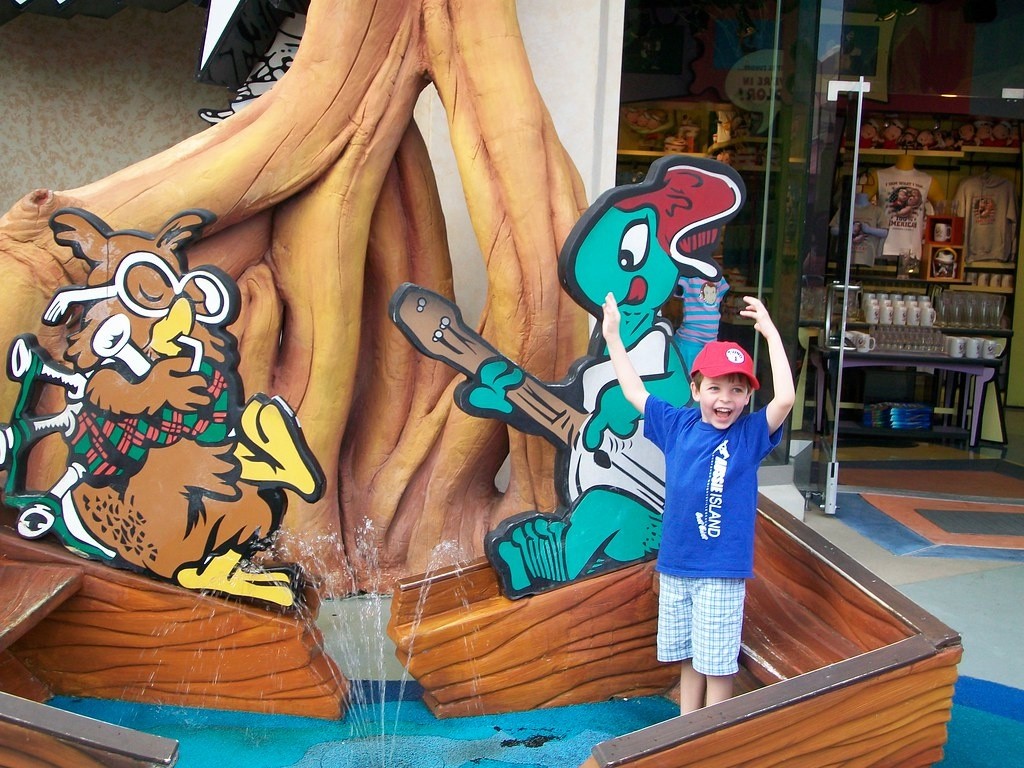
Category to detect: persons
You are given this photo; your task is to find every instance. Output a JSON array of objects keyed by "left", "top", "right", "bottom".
[
  {"left": 861, "top": 153, "right": 946, "bottom": 260},
  {"left": 830, "top": 193, "right": 889, "bottom": 269},
  {"left": 601, "top": 291, "right": 796, "bottom": 716}
]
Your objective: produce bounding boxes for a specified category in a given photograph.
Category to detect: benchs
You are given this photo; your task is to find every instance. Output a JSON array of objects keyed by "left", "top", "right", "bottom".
[
  {"left": 0, "top": 560, "right": 86, "bottom": 656},
  {"left": 653, "top": 563, "right": 869, "bottom": 688}
]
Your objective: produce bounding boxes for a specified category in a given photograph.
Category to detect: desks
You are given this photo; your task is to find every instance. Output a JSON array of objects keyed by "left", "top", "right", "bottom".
[{"left": 815, "top": 345, "right": 1003, "bottom": 448}]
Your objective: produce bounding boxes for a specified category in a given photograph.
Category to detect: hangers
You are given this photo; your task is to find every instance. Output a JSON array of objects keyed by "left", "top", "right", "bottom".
[{"left": 982, "top": 159, "right": 992, "bottom": 179}]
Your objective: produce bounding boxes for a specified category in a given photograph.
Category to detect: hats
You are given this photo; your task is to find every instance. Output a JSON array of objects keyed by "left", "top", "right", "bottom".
[{"left": 690, "top": 341, "right": 760, "bottom": 392}]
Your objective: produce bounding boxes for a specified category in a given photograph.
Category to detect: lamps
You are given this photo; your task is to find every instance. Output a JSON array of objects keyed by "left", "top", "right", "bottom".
[{"left": 876, "top": 0, "right": 919, "bottom": 21}]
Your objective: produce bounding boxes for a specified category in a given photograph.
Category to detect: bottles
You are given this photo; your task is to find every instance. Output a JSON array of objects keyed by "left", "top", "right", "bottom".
[{"left": 869, "top": 326, "right": 944, "bottom": 353}]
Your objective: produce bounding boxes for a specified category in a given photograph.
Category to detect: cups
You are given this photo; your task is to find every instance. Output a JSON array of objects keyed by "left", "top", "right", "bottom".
[
  {"left": 845, "top": 330, "right": 876, "bottom": 353},
  {"left": 934, "top": 290, "right": 1006, "bottom": 330},
  {"left": 879, "top": 306, "right": 893, "bottom": 325},
  {"left": 944, "top": 336, "right": 986, "bottom": 359},
  {"left": 865, "top": 304, "right": 879, "bottom": 325},
  {"left": 907, "top": 307, "right": 920, "bottom": 325},
  {"left": 935, "top": 223, "right": 951, "bottom": 241},
  {"left": 861, "top": 293, "right": 933, "bottom": 320},
  {"left": 920, "top": 301, "right": 936, "bottom": 325},
  {"left": 893, "top": 307, "right": 906, "bottom": 325},
  {"left": 964, "top": 272, "right": 1013, "bottom": 288},
  {"left": 983, "top": 340, "right": 1002, "bottom": 359},
  {"left": 897, "top": 249, "right": 910, "bottom": 280}
]
